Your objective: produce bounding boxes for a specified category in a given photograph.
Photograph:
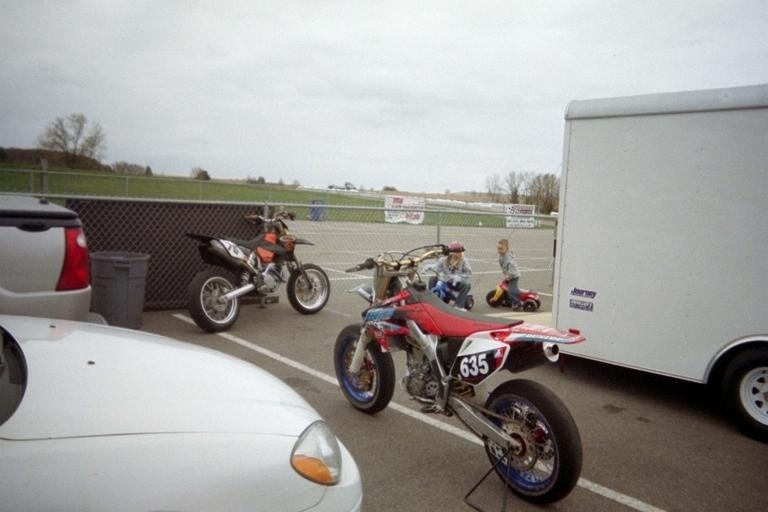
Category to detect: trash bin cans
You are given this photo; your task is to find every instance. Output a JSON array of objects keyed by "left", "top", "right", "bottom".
[
  {"left": 311, "top": 200, "right": 324, "bottom": 220},
  {"left": 90, "top": 252, "right": 150, "bottom": 328}
]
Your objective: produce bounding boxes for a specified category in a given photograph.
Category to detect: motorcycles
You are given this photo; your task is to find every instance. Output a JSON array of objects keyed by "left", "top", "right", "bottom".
[
  {"left": 180, "top": 212, "right": 331, "bottom": 332},
  {"left": 334, "top": 245, "right": 585, "bottom": 512}
]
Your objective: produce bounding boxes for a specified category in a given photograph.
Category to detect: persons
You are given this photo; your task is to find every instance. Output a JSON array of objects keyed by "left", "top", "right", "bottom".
[
  {"left": 423, "top": 241, "right": 472, "bottom": 309},
  {"left": 497, "top": 238, "right": 521, "bottom": 310}
]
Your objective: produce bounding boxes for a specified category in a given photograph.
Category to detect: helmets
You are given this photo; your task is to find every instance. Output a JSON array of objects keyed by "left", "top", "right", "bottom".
[{"left": 448, "top": 241, "right": 464, "bottom": 252}]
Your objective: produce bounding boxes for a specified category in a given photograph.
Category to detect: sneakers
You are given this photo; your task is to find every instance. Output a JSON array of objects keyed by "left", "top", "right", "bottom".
[
  {"left": 512, "top": 302, "right": 520, "bottom": 310},
  {"left": 455, "top": 305, "right": 467, "bottom": 312}
]
